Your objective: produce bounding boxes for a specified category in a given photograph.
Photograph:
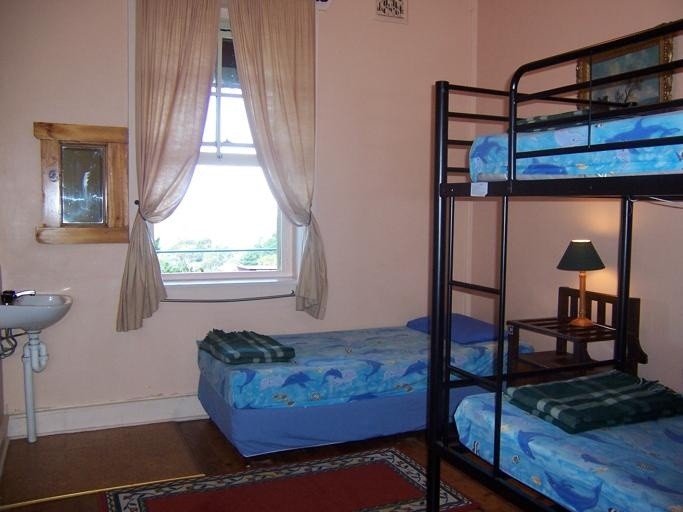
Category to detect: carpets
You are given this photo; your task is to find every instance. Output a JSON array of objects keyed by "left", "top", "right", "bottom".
[{"left": 98, "top": 445, "right": 480, "bottom": 511}]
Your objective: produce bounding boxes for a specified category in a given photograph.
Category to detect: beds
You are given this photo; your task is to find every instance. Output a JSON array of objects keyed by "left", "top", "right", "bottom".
[
  {"left": 196, "top": 317, "right": 534, "bottom": 458},
  {"left": 426, "top": 20, "right": 681, "bottom": 512}
]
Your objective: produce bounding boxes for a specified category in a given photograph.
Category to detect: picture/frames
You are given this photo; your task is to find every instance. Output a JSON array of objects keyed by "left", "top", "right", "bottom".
[{"left": 575, "top": 22, "right": 673, "bottom": 108}]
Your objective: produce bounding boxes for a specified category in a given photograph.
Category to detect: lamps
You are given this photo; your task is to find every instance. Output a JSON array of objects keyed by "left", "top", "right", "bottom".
[{"left": 555, "top": 238, "right": 605, "bottom": 327}]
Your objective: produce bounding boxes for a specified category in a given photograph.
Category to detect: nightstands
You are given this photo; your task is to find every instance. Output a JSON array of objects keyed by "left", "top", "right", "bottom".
[{"left": 505, "top": 285, "right": 648, "bottom": 387}]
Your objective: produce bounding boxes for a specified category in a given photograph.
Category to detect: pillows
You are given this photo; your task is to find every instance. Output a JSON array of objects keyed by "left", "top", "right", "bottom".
[{"left": 406, "top": 313, "right": 508, "bottom": 346}]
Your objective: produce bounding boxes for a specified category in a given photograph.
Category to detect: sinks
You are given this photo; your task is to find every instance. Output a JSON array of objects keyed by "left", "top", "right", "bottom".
[{"left": 0, "top": 294, "right": 73, "bottom": 328}]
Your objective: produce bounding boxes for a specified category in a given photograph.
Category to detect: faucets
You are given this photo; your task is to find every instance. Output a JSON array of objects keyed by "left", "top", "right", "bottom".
[{"left": 1, "top": 289, "right": 37, "bottom": 303}]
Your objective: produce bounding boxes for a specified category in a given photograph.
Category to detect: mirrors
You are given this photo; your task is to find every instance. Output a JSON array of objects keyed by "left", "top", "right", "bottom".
[{"left": 31, "top": 124, "right": 130, "bottom": 246}]
